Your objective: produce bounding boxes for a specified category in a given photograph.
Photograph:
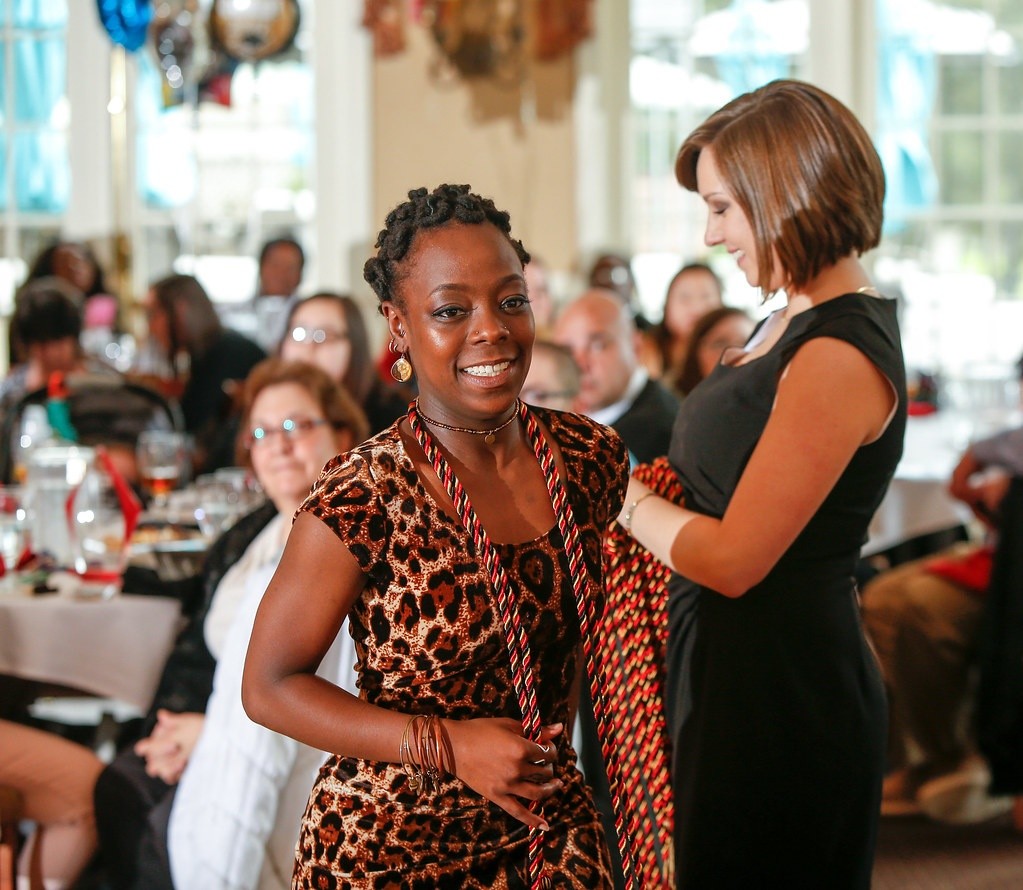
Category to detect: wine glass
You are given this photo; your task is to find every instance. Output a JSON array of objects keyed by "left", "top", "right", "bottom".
[{"left": 137, "top": 430, "right": 186, "bottom": 511}]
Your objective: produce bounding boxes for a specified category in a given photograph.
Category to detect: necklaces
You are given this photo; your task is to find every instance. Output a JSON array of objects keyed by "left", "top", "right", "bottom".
[
  {"left": 781, "top": 285, "right": 877, "bottom": 321},
  {"left": 414, "top": 395, "right": 520, "bottom": 445}
]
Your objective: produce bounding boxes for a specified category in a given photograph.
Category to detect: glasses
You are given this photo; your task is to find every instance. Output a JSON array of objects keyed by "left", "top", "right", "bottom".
[
  {"left": 286, "top": 324, "right": 350, "bottom": 348},
  {"left": 243, "top": 414, "right": 328, "bottom": 450}
]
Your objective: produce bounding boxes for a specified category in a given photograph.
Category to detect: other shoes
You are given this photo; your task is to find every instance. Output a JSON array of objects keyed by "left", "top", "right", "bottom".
[
  {"left": 878, "top": 769, "right": 922, "bottom": 816},
  {"left": 914, "top": 759, "right": 1015, "bottom": 826}
]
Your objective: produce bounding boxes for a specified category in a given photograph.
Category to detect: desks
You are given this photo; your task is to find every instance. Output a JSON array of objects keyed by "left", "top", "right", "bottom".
[
  {"left": 1, "top": 582, "right": 183, "bottom": 721},
  {"left": 867, "top": 460, "right": 986, "bottom": 546}
]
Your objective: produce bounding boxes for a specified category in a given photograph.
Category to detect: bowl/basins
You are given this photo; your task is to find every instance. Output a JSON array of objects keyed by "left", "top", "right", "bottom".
[{"left": 152, "top": 539, "right": 204, "bottom": 583}]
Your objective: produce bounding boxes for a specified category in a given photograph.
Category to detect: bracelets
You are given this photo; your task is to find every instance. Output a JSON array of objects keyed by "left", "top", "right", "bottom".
[
  {"left": 625, "top": 493, "right": 657, "bottom": 533},
  {"left": 399, "top": 714, "right": 445, "bottom": 799}
]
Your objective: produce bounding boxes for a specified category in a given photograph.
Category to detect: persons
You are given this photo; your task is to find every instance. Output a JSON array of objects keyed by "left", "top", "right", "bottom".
[
  {"left": 521, "top": 253, "right": 1023, "bottom": 828},
  {"left": 239, "top": 184, "right": 630, "bottom": 890},
  {"left": 0, "top": 236, "right": 410, "bottom": 890},
  {"left": 616, "top": 78, "right": 907, "bottom": 890}
]
